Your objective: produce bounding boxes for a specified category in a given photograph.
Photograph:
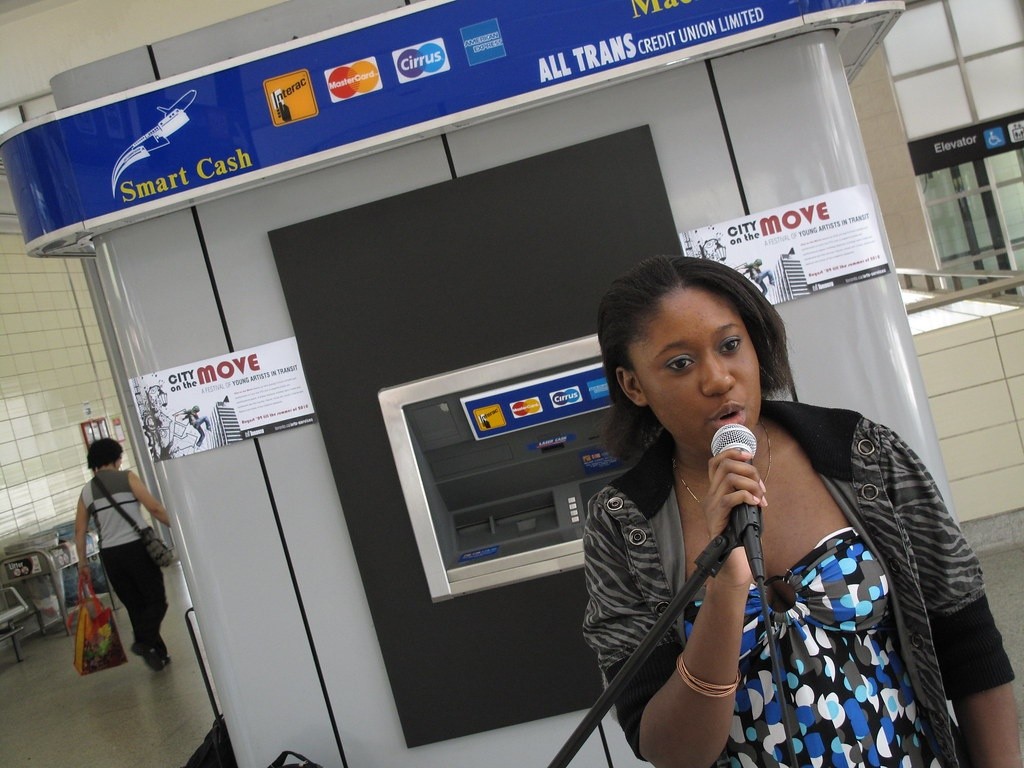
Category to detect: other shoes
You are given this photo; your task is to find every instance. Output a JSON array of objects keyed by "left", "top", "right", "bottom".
[{"left": 131, "top": 643, "right": 170, "bottom": 670}]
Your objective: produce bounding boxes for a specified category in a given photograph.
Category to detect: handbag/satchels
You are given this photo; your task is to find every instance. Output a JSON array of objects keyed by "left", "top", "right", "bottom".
[
  {"left": 68, "top": 575, "right": 128, "bottom": 675},
  {"left": 141, "top": 526, "right": 173, "bottom": 568}
]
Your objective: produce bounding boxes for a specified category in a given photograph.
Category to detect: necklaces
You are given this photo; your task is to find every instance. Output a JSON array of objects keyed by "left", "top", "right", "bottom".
[{"left": 672, "top": 420, "right": 772, "bottom": 505}]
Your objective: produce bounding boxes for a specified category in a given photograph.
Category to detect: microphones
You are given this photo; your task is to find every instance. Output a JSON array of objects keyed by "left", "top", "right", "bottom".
[{"left": 711, "top": 423, "right": 765, "bottom": 580}]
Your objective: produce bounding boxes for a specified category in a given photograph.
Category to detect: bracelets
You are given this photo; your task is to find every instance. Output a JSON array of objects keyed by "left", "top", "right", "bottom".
[
  {"left": 77, "top": 562, "right": 89, "bottom": 568},
  {"left": 676, "top": 651, "right": 741, "bottom": 698}
]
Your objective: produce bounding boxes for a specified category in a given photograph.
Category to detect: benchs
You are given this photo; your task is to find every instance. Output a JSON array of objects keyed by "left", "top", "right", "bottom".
[{"left": 0, "top": 587, "right": 28, "bottom": 663}]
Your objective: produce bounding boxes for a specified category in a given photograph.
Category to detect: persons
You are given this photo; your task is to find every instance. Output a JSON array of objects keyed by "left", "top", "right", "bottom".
[
  {"left": 581, "top": 253, "right": 1022, "bottom": 768},
  {"left": 181, "top": 405, "right": 212, "bottom": 449},
  {"left": 75, "top": 438, "right": 172, "bottom": 671}
]
